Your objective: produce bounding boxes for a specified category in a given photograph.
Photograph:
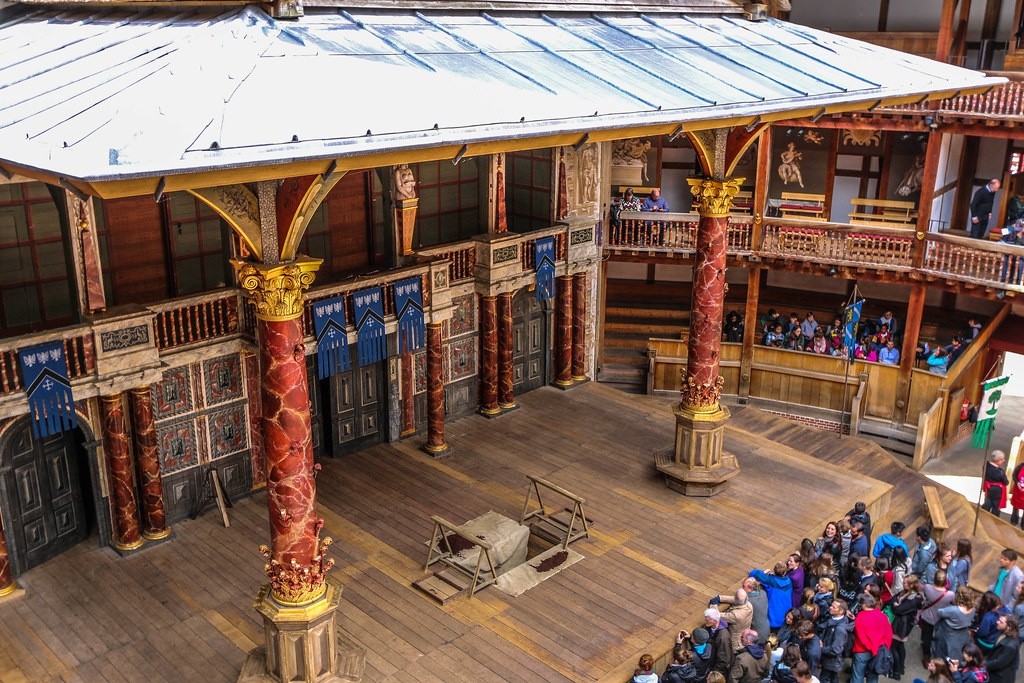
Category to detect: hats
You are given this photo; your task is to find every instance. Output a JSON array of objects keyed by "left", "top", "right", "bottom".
[
  {"left": 855, "top": 502, "right": 866, "bottom": 514},
  {"left": 807, "top": 312, "right": 815, "bottom": 317},
  {"left": 625, "top": 188, "right": 633, "bottom": 196},
  {"left": 693, "top": 627, "right": 709, "bottom": 644}
]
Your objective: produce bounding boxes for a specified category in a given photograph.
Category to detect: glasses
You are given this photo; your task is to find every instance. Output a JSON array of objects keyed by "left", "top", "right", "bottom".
[{"left": 788, "top": 641, "right": 799, "bottom": 648}]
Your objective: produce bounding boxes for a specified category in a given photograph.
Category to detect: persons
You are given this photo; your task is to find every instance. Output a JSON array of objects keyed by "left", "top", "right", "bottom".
[
  {"left": 394, "top": 164, "right": 416, "bottom": 200},
  {"left": 760, "top": 308, "right": 900, "bottom": 365},
  {"left": 618, "top": 188, "right": 641, "bottom": 244},
  {"left": 723, "top": 311, "right": 743, "bottom": 341},
  {"left": 634, "top": 501, "right": 1024, "bottom": 683},
  {"left": 1010, "top": 462, "right": 1024, "bottom": 528},
  {"left": 980, "top": 450, "right": 1009, "bottom": 517},
  {"left": 642, "top": 189, "right": 669, "bottom": 244},
  {"left": 916, "top": 316, "right": 982, "bottom": 375},
  {"left": 970, "top": 178, "right": 1000, "bottom": 238},
  {"left": 1001, "top": 191, "right": 1024, "bottom": 285}
]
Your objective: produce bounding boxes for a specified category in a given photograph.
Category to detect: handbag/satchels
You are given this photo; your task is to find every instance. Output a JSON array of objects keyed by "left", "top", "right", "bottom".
[
  {"left": 882, "top": 606, "right": 896, "bottom": 623},
  {"left": 914, "top": 609, "right": 923, "bottom": 625}
]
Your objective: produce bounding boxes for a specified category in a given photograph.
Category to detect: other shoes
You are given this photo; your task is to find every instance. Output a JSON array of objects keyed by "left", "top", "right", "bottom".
[
  {"left": 884, "top": 672, "right": 901, "bottom": 680},
  {"left": 901, "top": 667, "right": 905, "bottom": 675}
]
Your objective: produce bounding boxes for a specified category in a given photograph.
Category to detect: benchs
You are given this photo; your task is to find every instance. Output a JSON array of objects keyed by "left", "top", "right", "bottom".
[
  {"left": 619, "top": 186, "right": 661, "bottom": 206},
  {"left": 848, "top": 198, "right": 916, "bottom": 230},
  {"left": 690, "top": 190, "right": 827, "bottom": 222}
]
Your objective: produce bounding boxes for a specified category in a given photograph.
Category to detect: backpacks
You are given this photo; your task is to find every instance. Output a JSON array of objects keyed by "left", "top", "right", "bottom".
[{"left": 870, "top": 646, "right": 894, "bottom": 674}]
